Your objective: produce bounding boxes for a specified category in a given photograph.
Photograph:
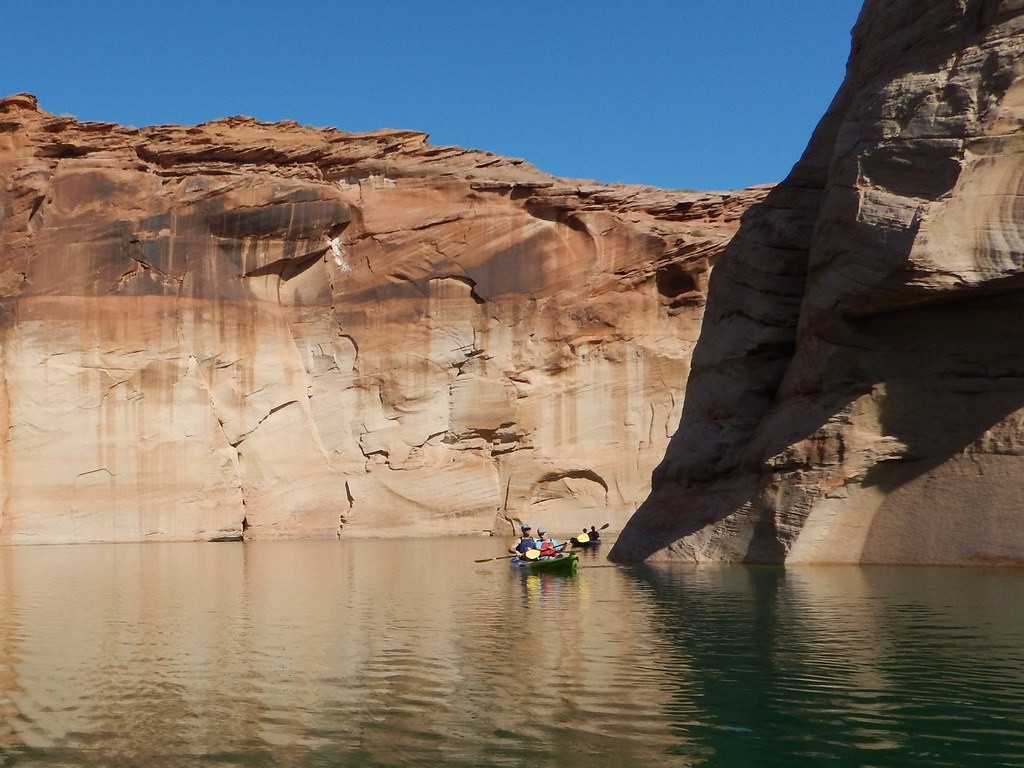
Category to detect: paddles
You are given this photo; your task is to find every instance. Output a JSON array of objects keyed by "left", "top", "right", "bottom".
[
  {"left": 474, "top": 533, "right": 590, "bottom": 563},
  {"left": 597, "top": 523, "right": 610, "bottom": 533}
]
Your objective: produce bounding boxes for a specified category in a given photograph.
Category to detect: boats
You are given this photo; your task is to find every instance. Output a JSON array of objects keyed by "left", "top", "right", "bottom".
[
  {"left": 571, "top": 538, "right": 601, "bottom": 547},
  {"left": 520, "top": 554, "right": 579, "bottom": 575}
]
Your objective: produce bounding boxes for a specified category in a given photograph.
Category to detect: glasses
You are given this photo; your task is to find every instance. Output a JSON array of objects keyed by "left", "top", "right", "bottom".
[
  {"left": 523, "top": 529, "right": 531, "bottom": 531},
  {"left": 539, "top": 533, "right": 545, "bottom": 536}
]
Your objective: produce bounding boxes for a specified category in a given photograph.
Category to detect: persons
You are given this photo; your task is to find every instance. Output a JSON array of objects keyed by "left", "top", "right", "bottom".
[
  {"left": 583, "top": 528, "right": 587, "bottom": 533},
  {"left": 508, "top": 524, "right": 553, "bottom": 560},
  {"left": 536, "top": 528, "right": 567, "bottom": 558},
  {"left": 588, "top": 526, "right": 599, "bottom": 541}
]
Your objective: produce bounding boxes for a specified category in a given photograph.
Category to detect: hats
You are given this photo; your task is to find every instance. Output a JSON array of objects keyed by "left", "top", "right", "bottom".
[
  {"left": 537, "top": 528, "right": 546, "bottom": 535},
  {"left": 521, "top": 524, "right": 531, "bottom": 531}
]
